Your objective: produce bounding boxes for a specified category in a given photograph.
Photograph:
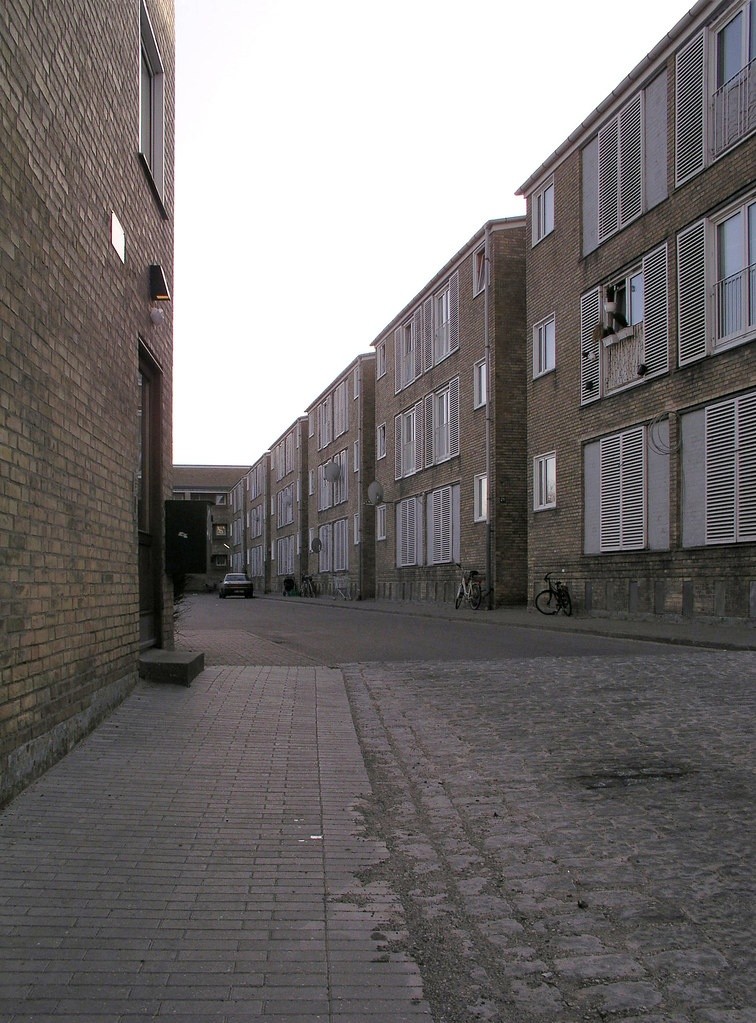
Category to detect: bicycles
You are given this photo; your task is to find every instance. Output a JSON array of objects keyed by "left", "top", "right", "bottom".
[
  {"left": 454, "top": 562, "right": 482, "bottom": 610},
  {"left": 299, "top": 572, "right": 316, "bottom": 598},
  {"left": 534, "top": 571, "right": 573, "bottom": 617}
]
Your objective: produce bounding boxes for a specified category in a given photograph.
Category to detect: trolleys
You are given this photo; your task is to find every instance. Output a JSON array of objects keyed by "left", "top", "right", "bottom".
[{"left": 330, "top": 570, "right": 352, "bottom": 601}]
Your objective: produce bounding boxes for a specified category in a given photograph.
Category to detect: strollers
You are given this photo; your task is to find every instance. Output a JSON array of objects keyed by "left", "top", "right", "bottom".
[{"left": 283, "top": 576, "right": 299, "bottom": 596}]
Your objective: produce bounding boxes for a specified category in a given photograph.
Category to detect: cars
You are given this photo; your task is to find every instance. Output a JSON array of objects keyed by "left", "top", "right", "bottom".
[{"left": 218, "top": 572, "right": 254, "bottom": 598}]
